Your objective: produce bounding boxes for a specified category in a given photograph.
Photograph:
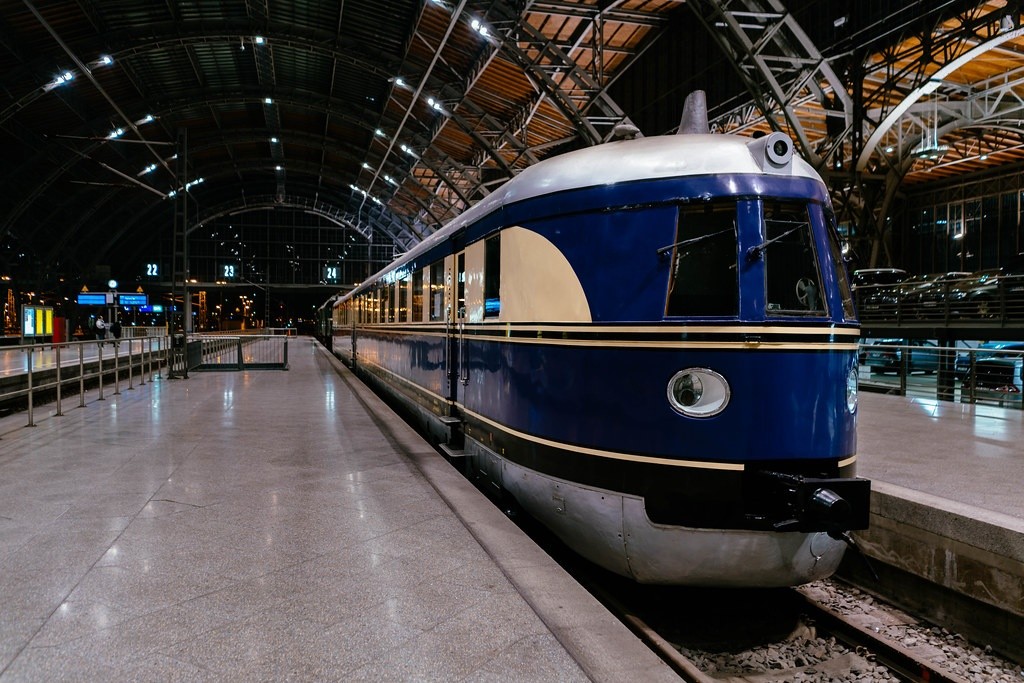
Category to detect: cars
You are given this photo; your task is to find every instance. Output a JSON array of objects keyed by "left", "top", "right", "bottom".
[
  {"left": 859, "top": 336, "right": 1024, "bottom": 390},
  {"left": 850, "top": 267, "right": 1024, "bottom": 320}
]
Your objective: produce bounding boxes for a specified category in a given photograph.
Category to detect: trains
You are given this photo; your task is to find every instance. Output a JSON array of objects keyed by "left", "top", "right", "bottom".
[{"left": 331, "top": 88, "right": 872, "bottom": 588}]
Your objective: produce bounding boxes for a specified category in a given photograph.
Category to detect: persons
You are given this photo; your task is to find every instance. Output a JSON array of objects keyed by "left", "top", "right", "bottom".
[
  {"left": 94, "top": 316, "right": 105, "bottom": 348},
  {"left": 109, "top": 322, "right": 122, "bottom": 348}
]
